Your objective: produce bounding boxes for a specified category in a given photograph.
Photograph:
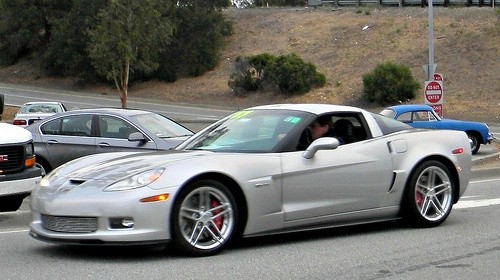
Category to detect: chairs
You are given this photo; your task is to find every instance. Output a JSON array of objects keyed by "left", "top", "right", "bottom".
[
  {"left": 119, "top": 122, "right": 133, "bottom": 139},
  {"left": 334, "top": 119, "right": 358, "bottom": 144},
  {"left": 415, "top": 113, "right": 420, "bottom": 121},
  {"left": 99, "top": 120, "right": 109, "bottom": 137}
]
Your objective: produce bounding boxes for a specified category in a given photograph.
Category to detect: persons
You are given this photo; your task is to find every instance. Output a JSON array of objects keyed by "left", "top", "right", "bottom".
[{"left": 274, "top": 114, "right": 333, "bottom": 151}]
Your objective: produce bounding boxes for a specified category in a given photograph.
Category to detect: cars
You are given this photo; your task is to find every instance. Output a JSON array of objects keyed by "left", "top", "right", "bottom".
[
  {"left": 374, "top": 103, "right": 493, "bottom": 155},
  {"left": 13, "top": 101, "right": 66, "bottom": 129}
]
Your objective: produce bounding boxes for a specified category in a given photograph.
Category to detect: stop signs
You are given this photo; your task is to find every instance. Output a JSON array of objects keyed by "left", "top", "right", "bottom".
[{"left": 424, "top": 80, "right": 443, "bottom": 105}]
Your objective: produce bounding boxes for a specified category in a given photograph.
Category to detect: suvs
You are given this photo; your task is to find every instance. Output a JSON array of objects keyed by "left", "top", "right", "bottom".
[{"left": 0, "top": 96, "right": 46, "bottom": 213}]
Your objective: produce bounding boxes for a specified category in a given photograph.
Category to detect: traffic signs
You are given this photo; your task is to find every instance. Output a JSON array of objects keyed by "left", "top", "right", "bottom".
[
  {"left": 433, "top": 74, "right": 443, "bottom": 82},
  {"left": 426, "top": 104, "right": 444, "bottom": 120}
]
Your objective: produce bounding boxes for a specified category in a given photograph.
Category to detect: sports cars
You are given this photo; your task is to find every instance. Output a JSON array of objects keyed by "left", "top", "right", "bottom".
[
  {"left": 29, "top": 101, "right": 477, "bottom": 254},
  {"left": 19, "top": 108, "right": 198, "bottom": 171}
]
